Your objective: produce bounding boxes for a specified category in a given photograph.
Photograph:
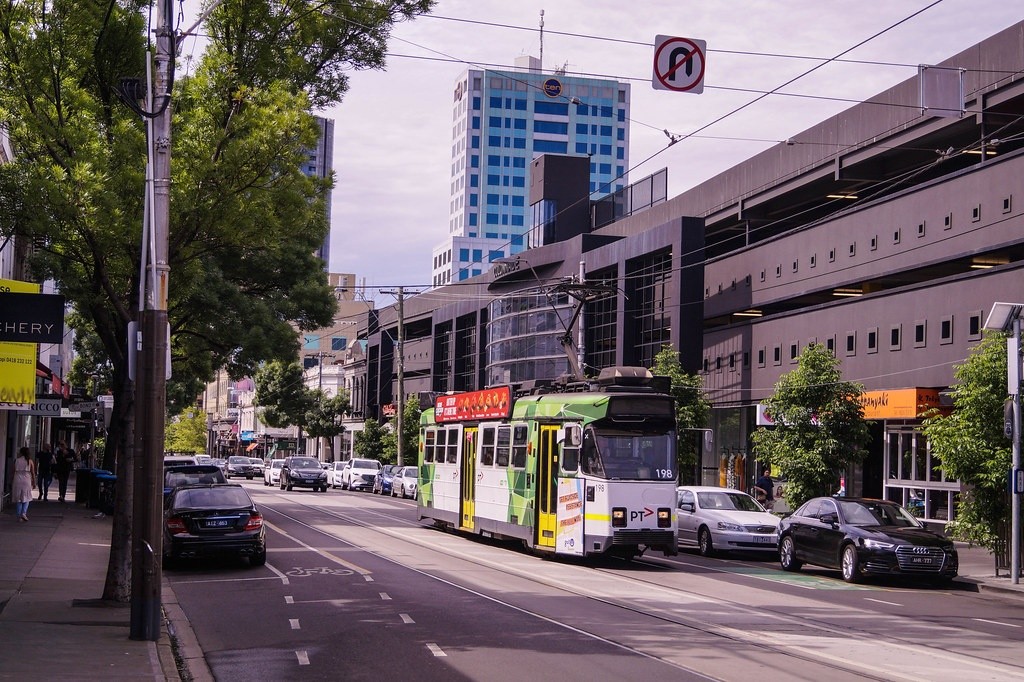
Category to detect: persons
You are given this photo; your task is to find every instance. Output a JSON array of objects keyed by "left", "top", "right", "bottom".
[
  {"left": 56, "top": 440, "right": 77, "bottom": 503},
  {"left": 720, "top": 453, "right": 747, "bottom": 492},
  {"left": 588, "top": 447, "right": 599, "bottom": 474},
  {"left": 49, "top": 443, "right": 90, "bottom": 480},
  {"left": 774, "top": 486, "right": 783, "bottom": 499},
  {"left": 10, "top": 447, "right": 35, "bottom": 523},
  {"left": 36, "top": 442, "right": 55, "bottom": 502},
  {"left": 754, "top": 468, "right": 774, "bottom": 511}
]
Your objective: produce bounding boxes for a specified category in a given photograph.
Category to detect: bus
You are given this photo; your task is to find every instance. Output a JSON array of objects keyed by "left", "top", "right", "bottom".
[{"left": 417, "top": 367, "right": 714, "bottom": 560}]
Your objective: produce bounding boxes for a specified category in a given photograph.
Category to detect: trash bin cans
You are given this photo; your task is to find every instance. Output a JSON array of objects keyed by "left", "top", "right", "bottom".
[{"left": 86, "top": 469, "right": 117, "bottom": 515}]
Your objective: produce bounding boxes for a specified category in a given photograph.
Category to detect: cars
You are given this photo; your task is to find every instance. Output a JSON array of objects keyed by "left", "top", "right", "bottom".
[
  {"left": 162, "top": 483, "right": 266, "bottom": 569},
  {"left": 164, "top": 448, "right": 263, "bottom": 492},
  {"left": 319, "top": 457, "right": 420, "bottom": 499},
  {"left": 777, "top": 497, "right": 959, "bottom": 587},
  {"left": 677, "top": 485, "right": 781, "bottom": 558},
  {"left": 265, "top": 459, "right": 285, "bottom": 486},
  {"left": 279, "top": 455, "right": 328, "bottom": 493}
]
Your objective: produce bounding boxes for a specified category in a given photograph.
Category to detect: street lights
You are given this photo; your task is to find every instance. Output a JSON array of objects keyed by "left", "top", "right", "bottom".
[{"left": 335, "top": 287, "right": 403, "bottom": 464}]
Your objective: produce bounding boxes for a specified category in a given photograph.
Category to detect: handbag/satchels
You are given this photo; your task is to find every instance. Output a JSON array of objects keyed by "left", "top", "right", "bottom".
[{"left": 757, "top": 494, "right": 766, "bottom": 503}]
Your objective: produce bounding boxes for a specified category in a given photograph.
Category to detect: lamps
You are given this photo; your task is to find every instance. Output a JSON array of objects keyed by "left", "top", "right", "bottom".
[{"left": 786, "top": 139, "right": 796, "bottom": 145}]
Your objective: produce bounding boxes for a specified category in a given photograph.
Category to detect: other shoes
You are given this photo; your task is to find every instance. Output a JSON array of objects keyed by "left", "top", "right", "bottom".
[
  {"left": 21, "top": 513, "right": 28, "bottom": 521},
  {"left": 18, "top": 517, "right": 22, "bottom": 521},
  {"left": 38, "top": 492, "right": 43, "bottom": 500},
  {"left": 58, "top": 497, "right": 65, "bottom": 502},
  {"left": 44, "top": 496, "right": 48, "bottom": 501}
]
